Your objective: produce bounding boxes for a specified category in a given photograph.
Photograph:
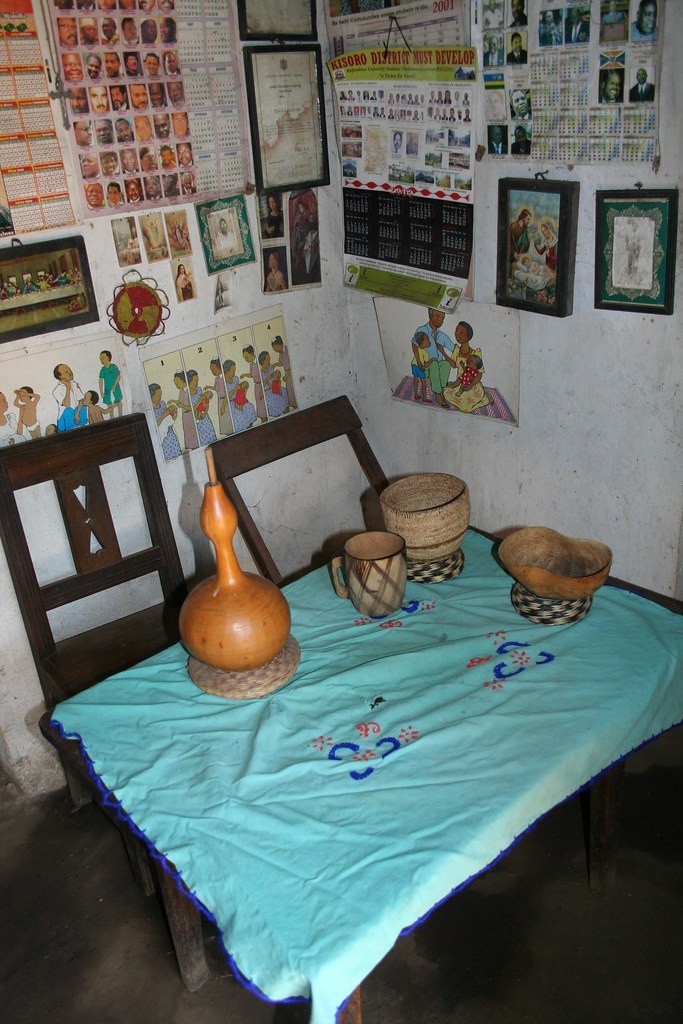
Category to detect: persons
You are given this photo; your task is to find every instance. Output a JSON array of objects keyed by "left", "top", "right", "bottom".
[
  {"left": 489, "top": 126, "right": 507, "bottom": 155},
  {"left": 483, "top": 36, "right": 502, "bottom": 64},
  {"left": 510, "top": 90, "right": 531, "bottom": 120},
  {"left": 603, "top": 0, "right": 626, "bottom": 24},
  {"left": 539, "top": 9, "right": 590, "bottom": 44},
  {"left": 483, "top": 0, "right": 503, "bottom": 27},
  {"left": 598, "top": 71, "right": 620, "bottom": 102},
  {"left": 339, "top": 89, "right": 471, "bottom": 122},
  {"left": 507, "top": 33, "right": 527, "bottom": 63},
  {"left": 632, "top": 0, "right": 657, "bottom": 40},
  {"left": 511, "top": 126, "right": 531, "bottom": 154},
  {"left": 489, "top": 91, "right": 505, "bottom": 120},
  {"left": 176, "top": 264, "right": 194, "bottom": 301},
  {"left": 393, "top": 132, "right": 403, "bottom": 152},
  {"left": 507, "top": 0, "right": 527, "bottom": 26},
  {"left": 625, "top": 223, "right": 640, "bottom": 277},
  {"left": 629, "top": 69, "right": 654, "bottom": 102},
  {"left": 53, "top": 0, "right": 194, "bottom": 207}
]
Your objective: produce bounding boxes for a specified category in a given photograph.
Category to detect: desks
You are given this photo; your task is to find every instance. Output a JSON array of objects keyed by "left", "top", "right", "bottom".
[{"left": 39, "top": 528, "right": 683, "bottom": 1024}]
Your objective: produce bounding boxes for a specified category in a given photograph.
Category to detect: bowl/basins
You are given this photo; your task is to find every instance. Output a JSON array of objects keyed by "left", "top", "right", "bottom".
[
  {"left": 498, "top": 526, "right": 613, "bottom": 599},
  {"left": 379, "top": 473, "right": 472, "bottom": 559}
]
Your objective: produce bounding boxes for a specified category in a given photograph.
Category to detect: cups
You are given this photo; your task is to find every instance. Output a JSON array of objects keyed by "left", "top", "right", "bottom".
[{"left": 332, "top": 531, "right": 407, "bottom": 618}]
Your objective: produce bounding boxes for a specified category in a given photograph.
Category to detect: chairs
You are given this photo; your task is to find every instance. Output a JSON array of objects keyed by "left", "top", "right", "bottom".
[
  {"left": 0, "top": 412, "right": 190, "bottom": 898},
  {"left": 207, "top": 395, "right": 391, "bottom": 589}
]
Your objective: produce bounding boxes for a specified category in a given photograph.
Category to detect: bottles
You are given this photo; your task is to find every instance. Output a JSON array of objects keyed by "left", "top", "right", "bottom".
[{"left": 177, "top": 482, "right": 291, "bottom": 674}]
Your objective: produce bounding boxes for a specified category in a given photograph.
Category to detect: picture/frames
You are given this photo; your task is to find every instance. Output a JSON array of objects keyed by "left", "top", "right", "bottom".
[
  {"left": 236, "top": 0, "right": 318, "bottom": 43},
  {"left": 594, "top": 189, "right": 678, "bottom": 315},
  {"left": 0, "top": 235, "right": 100, "bottom": 344},
  {"left": 243, "top": 44, "right": 330, "bottom": 197},
  {"left": 496, "top": 177, "right": 580, "bottom": 317}
]
